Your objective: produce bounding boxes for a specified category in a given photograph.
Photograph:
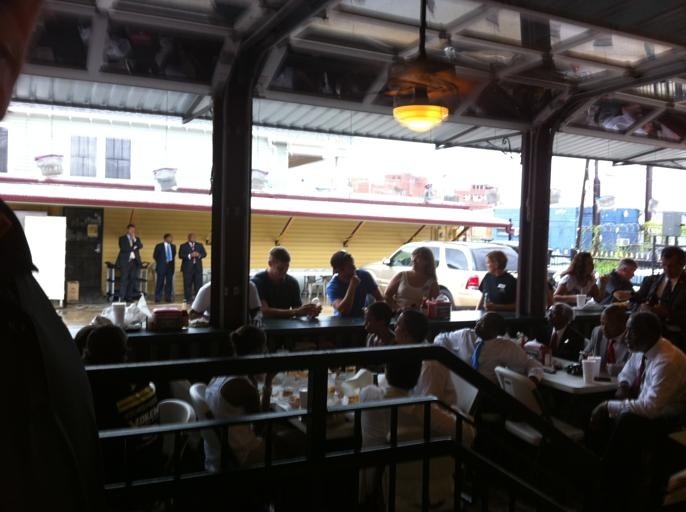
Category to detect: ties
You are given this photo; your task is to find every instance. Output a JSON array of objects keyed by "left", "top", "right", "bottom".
[
  {"left": 167, "top": 246, "right": 171, "bottom": 263},
  {"left": 606, "top": 340, "right": 618, "bottom": 363},
  {"left": 132, "top": 237, "right": 134, "bottom": 247},
  {"left": 548, "top": 330, "right": 559, "bottom": 354},
  {"left": 629, "top": 356, "right": 647, "bottom": 400},
  {"left": 471, "top": 341, "right": 485, "bottom": 370},
  {"left": 192, "top": 244, "right": 195, "bottom": 262},
  {"left": 659, "top": 280, "right": 674, "bottom": 306}
]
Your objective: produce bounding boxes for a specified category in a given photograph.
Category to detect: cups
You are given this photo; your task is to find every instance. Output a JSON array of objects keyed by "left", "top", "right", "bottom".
[
  {"left": 112, "top": 302, "right": 126, "bottom": 328},
  {"left": 582, "top": 359, "right": 596, "bottom": 385},
  {"left": 299, "top": 387, "right": 307, "bottom": 410},
  {"left": 577, "top": 294, "right": 587, "bottom": 307},
  {"left": 587, "top": 355, "right": 602, "bottom": 378}
]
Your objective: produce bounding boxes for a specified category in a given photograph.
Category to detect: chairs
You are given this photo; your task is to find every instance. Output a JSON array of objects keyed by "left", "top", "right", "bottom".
[
  {"left": 189, "top": 380, "right": 232, "bottom": 460},
  {"left": 155, "top": 396, "right": 195, "bottom": 460},
  {"left": 449, "top": 373, "right": 476, "bottom": 413},
  {"left": 494, "top": 365, "right": 546, "bottom": 446},
  {"left": 167, "top": 376, "right": 194, "bottom": 401}
]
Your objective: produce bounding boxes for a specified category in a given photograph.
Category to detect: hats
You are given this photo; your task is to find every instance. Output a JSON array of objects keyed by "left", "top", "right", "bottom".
[{"left": 329, "top": 247, "right": 348, "bottom": 273}]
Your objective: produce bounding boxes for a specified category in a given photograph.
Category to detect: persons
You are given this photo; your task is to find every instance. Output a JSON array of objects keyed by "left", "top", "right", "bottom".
[
  {"left": 0, "top": 0, "right": 105, "bottom": 511},
  {"left": 111, "top": 224, "right": 145, "bottom": 306},
  {"left": 151, "top": 228, "right": 177, "bottom": 305},
  {"left": 75, "top": 246, "right": 686, "bottom": 488},
  {"left": 179, "top": 233, "right": 208, "bottom": 303}
]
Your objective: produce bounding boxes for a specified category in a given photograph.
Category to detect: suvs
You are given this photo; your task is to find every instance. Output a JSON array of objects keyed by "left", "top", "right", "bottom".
[{"left": 356, "top": 241, "right": 518, "bottom": 310}]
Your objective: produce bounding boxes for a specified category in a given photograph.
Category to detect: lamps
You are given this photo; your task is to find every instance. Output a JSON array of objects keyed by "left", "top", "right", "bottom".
[{"left": 390, "top": 2, "right": 451, "bottom": 134}]
